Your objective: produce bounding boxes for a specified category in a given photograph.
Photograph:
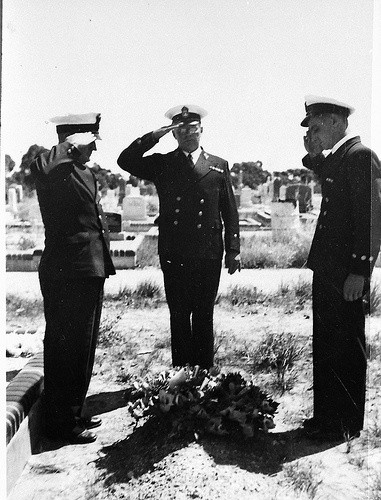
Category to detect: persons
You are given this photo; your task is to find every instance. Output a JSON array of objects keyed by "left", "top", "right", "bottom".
[
  {"left": 118, "top": 104, "right": 240, "bottom": 371},
  {"left": 301, "top": 95, "right": 381, "bottom": 440},
  {"left": 29, "top": 113, "right": 116, "bottom": 444}
]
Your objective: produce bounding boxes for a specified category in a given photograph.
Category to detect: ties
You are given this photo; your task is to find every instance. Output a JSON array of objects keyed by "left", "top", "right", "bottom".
[{"left": 188, "top": 154, "right": 194, "bottom": 166}]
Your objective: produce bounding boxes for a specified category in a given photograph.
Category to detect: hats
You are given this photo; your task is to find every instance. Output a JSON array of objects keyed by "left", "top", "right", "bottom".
[
  {"left": 300, "top": 95, "right": 355, "bottom": 126},
  {"left": 164, "top": 104, "right": 207, "bottom": 125},
  {"left": 50, "top": 112, "right": 102, "bottom": 141}
]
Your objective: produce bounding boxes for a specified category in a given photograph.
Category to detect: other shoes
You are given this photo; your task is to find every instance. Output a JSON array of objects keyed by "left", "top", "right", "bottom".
[
  {"left": 87, "top": 416, "right": 101, "bottom": 428},
  {"left": 68, "top": 429, "right": 97, "bottom": 443},
  {"left": 304, "top": 421, "right": 363, "bottom": 439}
]
